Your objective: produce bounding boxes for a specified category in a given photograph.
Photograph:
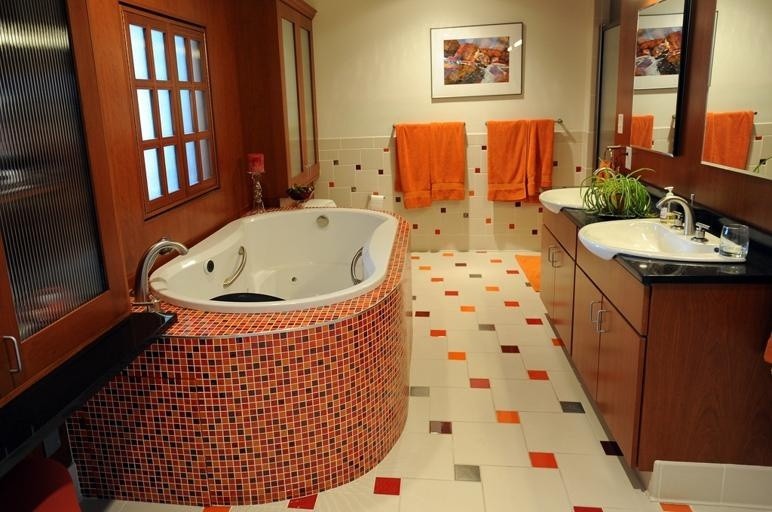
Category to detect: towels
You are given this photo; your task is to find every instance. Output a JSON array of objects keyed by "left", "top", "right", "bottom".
[
  {"left": 703, "top": 112, "right": 754, "bottom": 170},
  {"left": 487, "top": 119, "right": 555, "bottom": 204},
  {"left": 629, "top": 115, "right": 654, "bottom": 149},
  {"left": 395, "top": 122, "right": 465, "bottom": 210}
]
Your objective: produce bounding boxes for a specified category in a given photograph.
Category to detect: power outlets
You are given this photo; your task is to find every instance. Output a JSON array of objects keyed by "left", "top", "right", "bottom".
[{"left": 624, "top": 146, "right": 632, "bottom": 170}]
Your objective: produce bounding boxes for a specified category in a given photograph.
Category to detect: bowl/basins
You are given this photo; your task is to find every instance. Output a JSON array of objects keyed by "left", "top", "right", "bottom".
[{"left": 287, "top": 186, "right": 315, "bottom": 201}]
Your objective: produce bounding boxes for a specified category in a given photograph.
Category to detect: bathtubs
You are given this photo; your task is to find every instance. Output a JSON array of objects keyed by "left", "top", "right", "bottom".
[{"left": 149, "top": 206, "right": 400, "bottom": 312}]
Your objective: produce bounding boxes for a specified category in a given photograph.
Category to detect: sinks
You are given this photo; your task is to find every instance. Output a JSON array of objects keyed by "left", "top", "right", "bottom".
[
  {"left": 537, "top": 186, "right": 612, "bottom": 215},
  {"left": 576, "top": 214, "right": 749, "bottom": 264}
]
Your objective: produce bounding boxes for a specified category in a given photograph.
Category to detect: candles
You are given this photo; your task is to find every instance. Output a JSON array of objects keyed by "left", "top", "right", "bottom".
[{"left": 247, "top": 153, "right": 265, "bottom": 173}]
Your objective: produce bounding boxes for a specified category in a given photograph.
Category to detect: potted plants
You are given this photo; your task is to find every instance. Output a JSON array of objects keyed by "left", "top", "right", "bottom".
[{"left": 577, "top": 166, "right": 661, "bottom": 219}]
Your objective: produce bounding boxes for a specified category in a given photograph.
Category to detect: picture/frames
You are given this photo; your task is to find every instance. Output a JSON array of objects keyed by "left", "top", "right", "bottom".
[
  {"left": 633, "top": 13, "right": 684, "bottom": 91},
  {"left": 429, "top": 21, "right": 523, "bottom": 100}
]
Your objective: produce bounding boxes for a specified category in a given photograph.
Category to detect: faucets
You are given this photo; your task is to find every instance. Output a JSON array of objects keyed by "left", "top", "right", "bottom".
[
  {"left": 133, "top": 237, "right": 189, "bottom": 305},
  {"left": 656, "top": 195, "right": 697, "bottom": 236}
]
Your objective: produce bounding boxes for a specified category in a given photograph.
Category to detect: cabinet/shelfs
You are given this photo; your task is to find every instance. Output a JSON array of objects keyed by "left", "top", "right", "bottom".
[
  {"left": 236, "top": 0, "right": 320, "bottom": 199},
  {"left": 539, "top": 207, "right": 576, "bottom": 355},
  {"left": 571, "top": 237, "right": 772, "bottom": 472},
  {"left": 0, "top": 0, "right": 133, "bottom": 410}
]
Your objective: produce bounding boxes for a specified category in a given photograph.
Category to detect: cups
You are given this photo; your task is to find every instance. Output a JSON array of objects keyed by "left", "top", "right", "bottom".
[
  {"left": 720, "top": 263, "right": 747, "bottom": 277},
  {"left": 720, "top": 223, "right": 749, "bottom": 257}
]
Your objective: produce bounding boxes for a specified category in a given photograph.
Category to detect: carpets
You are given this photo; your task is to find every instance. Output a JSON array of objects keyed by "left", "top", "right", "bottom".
[{"left": 513, "top": 254, "right": 543, "bottom": 292}]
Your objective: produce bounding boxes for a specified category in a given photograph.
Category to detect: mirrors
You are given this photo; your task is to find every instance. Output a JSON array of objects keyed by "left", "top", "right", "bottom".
[
  {"left": 629, "top": 1, "right": 696, "bottom": 159},
  {"left": 699, "top": 0, "right": 772, "bottom": 183}
]
